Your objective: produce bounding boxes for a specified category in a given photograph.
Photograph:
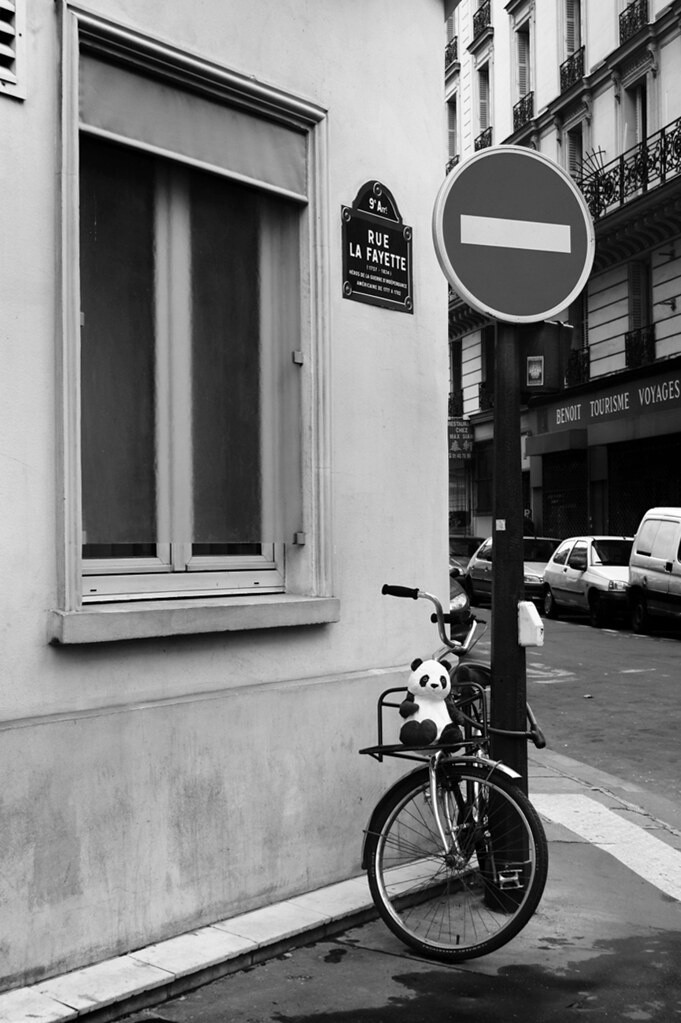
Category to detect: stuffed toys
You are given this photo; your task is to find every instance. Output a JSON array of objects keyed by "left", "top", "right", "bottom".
[{"left": 401, "top": 657, "right": 463, "bottom": 750}]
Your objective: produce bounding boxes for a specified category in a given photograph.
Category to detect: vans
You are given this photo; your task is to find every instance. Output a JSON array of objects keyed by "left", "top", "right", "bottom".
[{"left": 628, "top": 506, "right": 681, "bottom": 635}]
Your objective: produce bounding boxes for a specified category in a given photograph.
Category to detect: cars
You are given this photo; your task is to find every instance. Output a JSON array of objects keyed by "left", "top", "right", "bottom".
[
  {"left": 449, "top": 533, "right": 488, "bottom": 644},
  {"left": 540, "top": 535, "right": 635, "bottom": 625},
  {"left": 467, "top": 535, "right": 562, "bottom": 617}
]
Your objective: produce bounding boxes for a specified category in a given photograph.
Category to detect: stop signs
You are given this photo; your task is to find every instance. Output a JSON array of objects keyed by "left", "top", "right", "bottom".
[{"left": 433, "top": 144, "right": 595, "bottom": 325}]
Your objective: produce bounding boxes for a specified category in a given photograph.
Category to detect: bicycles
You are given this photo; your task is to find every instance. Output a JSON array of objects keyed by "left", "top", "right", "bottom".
[{"left": 358, "top": 583, "right": 548, "bottom": 965}]
